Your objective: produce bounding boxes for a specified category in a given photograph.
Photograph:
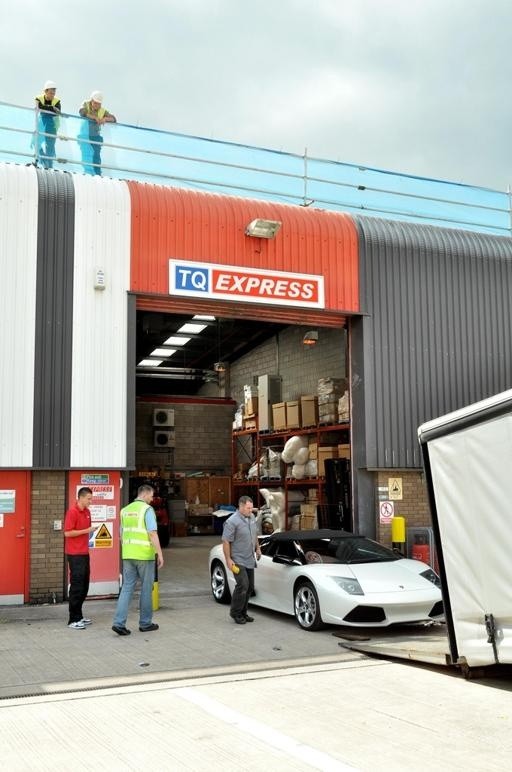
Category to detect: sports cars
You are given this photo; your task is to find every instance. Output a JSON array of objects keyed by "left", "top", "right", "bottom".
[{"left": 208, "top": 529, "right": 447, "bottom": 632}]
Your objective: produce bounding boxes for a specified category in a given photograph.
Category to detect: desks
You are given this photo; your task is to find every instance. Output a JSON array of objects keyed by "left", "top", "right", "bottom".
[{"left": 187, "top": 514, "right": 231, "bottom": 536}]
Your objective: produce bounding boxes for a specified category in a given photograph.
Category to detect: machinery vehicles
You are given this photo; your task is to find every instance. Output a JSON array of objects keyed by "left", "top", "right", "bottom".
[{"left": 130, "top": 465, "right": 169, "bottom": 547}]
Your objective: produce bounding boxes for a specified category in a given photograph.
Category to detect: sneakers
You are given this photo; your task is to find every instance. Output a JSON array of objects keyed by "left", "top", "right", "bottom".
[
  {"left": 243, "top": 614, "right": 254, "bottom": 621},
  {"left": 68, "top": 622, "right": 85, "bottom": 629},
  {"left": 139, "top": 623, "right": 159, "bottom": 631},
  {"left": 80, "top": 619, "right": 92, "bottom": 624},
  {"left": 112, "top": 626, "right": 131, "bottom": 635},
  {"left": 230, "top": 613, "right": 246, "bottom": 624}
]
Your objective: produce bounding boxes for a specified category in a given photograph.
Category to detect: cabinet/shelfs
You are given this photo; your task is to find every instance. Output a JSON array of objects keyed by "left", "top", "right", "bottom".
[
  {"left": 231, "top": 423, "right": 261, "bottom": 539},
  {"left": 317, "top": 422, "right": 353, "bottom": 534},
  {"left": 286, "top": 427, "right": 316, "bottom": 540},
  {"left": 258, "top": 427, "right": 286, "bottom": 543}
]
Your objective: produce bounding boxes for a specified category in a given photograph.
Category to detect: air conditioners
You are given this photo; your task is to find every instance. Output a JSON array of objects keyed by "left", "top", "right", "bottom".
[
  {"left": 153, "top": 409, "right": 174, "bottom": 426},
  {"left": 154, "top": 430, "right": 176, "bottom": 449}
]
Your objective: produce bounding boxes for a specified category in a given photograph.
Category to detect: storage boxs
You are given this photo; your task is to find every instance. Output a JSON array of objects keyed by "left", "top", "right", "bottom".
[
  {"left": 300, "top": 488, "right": 318, "bottom": 531},
  {"left": 337, "top": 443, "right": 350, "bottom": 463},
  {"left": 299, "top": 395, "right": 318, "bottom": 429},
  {"left": 232, "top": 404, "right": 258, "bottom": 432},
  {"left": 286, "top": 400, "right": 299, "bottom": 428},
  {"left": 271, "top": 400, "right": 287, "bottom": 431},
  {"left": 243, "top": 384, "right": 258, "bottom": 416},
  {"left": 167, "top": 499, "right": 187, "bottom": 537},
  {"left": 318, "top": 446, "right": 337, "bottom": 478},
  {"left": 307, "top": 443, "right": 319, "bottom": 461}
]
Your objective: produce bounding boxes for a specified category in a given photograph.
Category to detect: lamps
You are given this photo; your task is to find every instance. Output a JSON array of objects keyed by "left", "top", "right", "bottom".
[
  {"left": 303, "top": 329, "right": 318, "bottom": 345},
  {"left": 244, "top": 217, "right": 283, "bottom": 239},
  {"left": 214, "top": 322, "right": 227, "bottom": 372}
]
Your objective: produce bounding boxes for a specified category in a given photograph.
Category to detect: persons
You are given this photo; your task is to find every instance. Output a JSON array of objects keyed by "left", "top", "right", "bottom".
[
  {"left": 304, "top": 550, "right": 324, "bottom": 565},
  {"left": 29, "top": 80, "right": 63, "bottom": 170},
  {"left": 63, "top": 487, "right": 101, "bottom": 630},
  {"left": 221, "top": 495, "right": 262, "bottom": 625},
  {"left": 110, "top": 484, "right": 164, "bottom": 636},
  {"left": 75, "top": 90, "right": 117, "bottom": 176}
]
